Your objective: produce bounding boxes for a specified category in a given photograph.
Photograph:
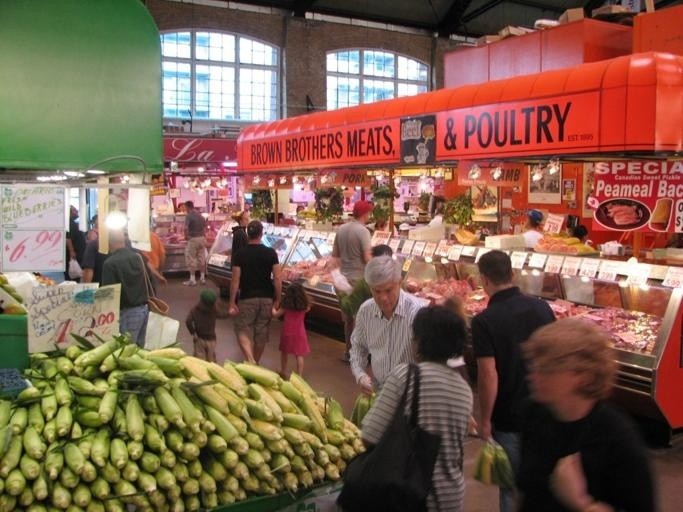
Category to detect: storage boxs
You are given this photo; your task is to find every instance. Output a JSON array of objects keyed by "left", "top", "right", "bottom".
[{"left": 0, "top": 309, "right": 40, "bottom": 379}]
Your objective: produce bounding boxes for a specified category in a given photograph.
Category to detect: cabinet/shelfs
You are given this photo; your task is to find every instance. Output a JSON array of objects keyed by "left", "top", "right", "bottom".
[
  {"left": 378, "top": 236, "right": 487, "bottom": 369},
  {"left": 204, "top": 215, "right": 299, "bottom": 303},
  {"left": 146, "top": 214, "right": 223, "bottom": 280},
  {"left": 489, "top": 245, "right": 683, "bottom": 452},
  {"left": 284, "top": 228, "right": 348, "bottom": 347}
]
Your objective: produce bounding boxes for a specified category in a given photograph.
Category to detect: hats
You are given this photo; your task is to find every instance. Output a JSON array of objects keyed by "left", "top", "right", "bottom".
[
  {"left": 352, "top": 201, "right": 373, "bottom": 215},
  {"left": 200, "top": 288, "right": 217, "bottom": 306}
]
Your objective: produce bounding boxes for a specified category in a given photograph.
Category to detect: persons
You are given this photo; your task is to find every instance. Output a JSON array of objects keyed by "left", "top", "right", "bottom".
[
  {"left": 361, "top": 305, "right": 475, "bottom": 510},
  {"left": 573, "top": 224, "right": 593, "bottom": 247},
  {"left": 523, "top": 208, "right": 544, "bottom": 244},
  {"left": 349, "top": 258, "right": 431, "bottom": 396},
  {"left": 520, "top": 319, "right": 658, "bottom": 511},
  {"left": 445, "top": 295, "right": 468, "bottom": 382},
  {"left": 471, "top": 250, "right": 557, "bottom": 510},
  {"left": 332, "top": 199, "right": 374, "bottom": 364},
  {"left": 428, "top": 208, "right": 446, "bottom": 227},
  {"left": 184, "top": 201, "right": 311, "bottom": 379},
  {"left": 66, "top": 204, "right": 165, "bottom": 349},
  {"left": 371, "top": 207, "right": 397, "bottom": 237},
  {"left": 340, "top": 246, "right": 393, "bottom": 317}
]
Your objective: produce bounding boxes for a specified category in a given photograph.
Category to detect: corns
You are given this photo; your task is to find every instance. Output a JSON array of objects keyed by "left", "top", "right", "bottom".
[
  {"left": 350, "top": 390, "right": 377, "bottom": 430},
  {"left": 472, "top": 445, "right": 514, "bottom": 487},
  {"left": 1, "top": 334, "right": 366, "bottom": 512}
]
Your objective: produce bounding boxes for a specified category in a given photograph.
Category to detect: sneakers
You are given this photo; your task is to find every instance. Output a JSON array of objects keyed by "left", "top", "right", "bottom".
[{"left": 183, "top": 280, "right": 197, "bottom": 286}]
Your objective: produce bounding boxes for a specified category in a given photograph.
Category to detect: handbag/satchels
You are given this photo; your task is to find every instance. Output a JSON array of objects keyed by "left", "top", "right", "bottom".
[
  {"left": 336, "top": 363, "right": 442, "bottom": 512},
  {"left": 137, "top": 253, "right": 170, "bottom": 315}
]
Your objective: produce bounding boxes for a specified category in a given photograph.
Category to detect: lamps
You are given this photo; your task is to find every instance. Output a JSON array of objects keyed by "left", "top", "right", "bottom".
[{"left": 465, "top": 155, "right": 560, "bottom": 183}]
